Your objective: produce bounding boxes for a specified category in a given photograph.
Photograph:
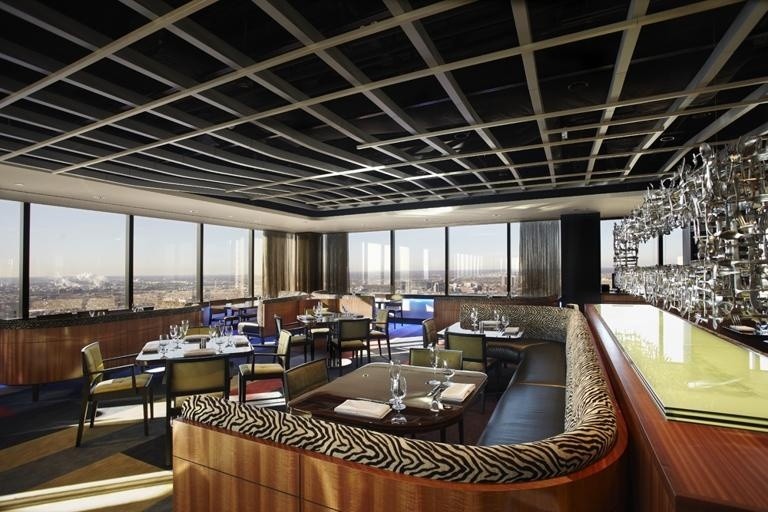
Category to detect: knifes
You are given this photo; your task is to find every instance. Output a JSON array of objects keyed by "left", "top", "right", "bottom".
[{"left": 426, "top": 385, "right": 441, "bottom": 397}]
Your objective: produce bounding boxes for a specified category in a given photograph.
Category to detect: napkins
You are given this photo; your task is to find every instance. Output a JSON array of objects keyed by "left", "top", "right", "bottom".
[
  {"left": 142, "top": 340, "right": 160, "bottom": 354},
  {"left": 183, "top": 347, "right": 216, "bottom": 357},
  {"left": 439, "top": 382, "right": 476, "bottom": 402},
  {"left": 334, "top": 399, "right": 393, "bottom": 421},
  {"left": 505, "top": 328, "right": 519, "bottom": 334},
  {"left": 185, "top": 334, "right": 212, "bottom": 343}
]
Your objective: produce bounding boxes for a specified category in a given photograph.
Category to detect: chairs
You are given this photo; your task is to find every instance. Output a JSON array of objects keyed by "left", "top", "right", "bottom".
[
  {"left": 422, "top": 317, "right": 445, "bottom": 349},
  {"left": 273, "top": 314, "right": 314, "bottom": 363},
  {"left": 330, "top": 319, "right": 369, "bottom": 377},
  {"left": 351, "top": 310, "right": 389, "bottom": 365},
  {"left": 75, "top": 290, "right": 406, "bottom": 329},
  {"left": 238, "top": 304, "right": 264, "bottom": 343},
  {"left": 445, "top": 330, "right": 497, "bottom": 403},
  {"left": 236, "top": 330, "right": 290, "bottom": 404},
  {"left": 76, "top": 342, "right": 155, "bottom": 449},
  {"left": 408, "top": 348, "right": 463, "bottom": 442},
  {"left": 162, "top": 327, "right": 218, "bottom": 390},
  {"left": 302, "top": 307, "right": 331, "bottom": 355}
]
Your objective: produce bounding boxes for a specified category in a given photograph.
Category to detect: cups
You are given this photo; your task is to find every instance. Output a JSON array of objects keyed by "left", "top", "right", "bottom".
[{"left": 312, "top": 301, "right": 322, "bottom": 319}]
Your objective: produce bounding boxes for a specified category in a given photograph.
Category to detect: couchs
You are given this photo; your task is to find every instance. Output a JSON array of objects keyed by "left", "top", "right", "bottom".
[{"left": 167, "top": 302, "right": 630, "bottom": 511}]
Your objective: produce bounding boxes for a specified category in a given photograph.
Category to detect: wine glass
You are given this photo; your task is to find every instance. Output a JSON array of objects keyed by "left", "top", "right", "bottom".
[
  {"left": 425, "top": 343, "right": 441, "bottom": 385},
  {"left": 342, "top": 304, "right": 350, "bottom": 318},
  {"left": 388, "top": 359, "right": 407, "bottom": 410},
  {"left": 208, "top": 324, "right": 233, "bottom": 355},
  {"left": 493, "top": 310, "right": 509, "bottom": 332},
  {"left": 159, "top": 319, "right": 189, "bottom": 359},
  {"left": 442, "top": 358, "right": 455, "bottom": 386},
  {"left": 470, "top": 307, "right": 478, "bottom": 326}
]
